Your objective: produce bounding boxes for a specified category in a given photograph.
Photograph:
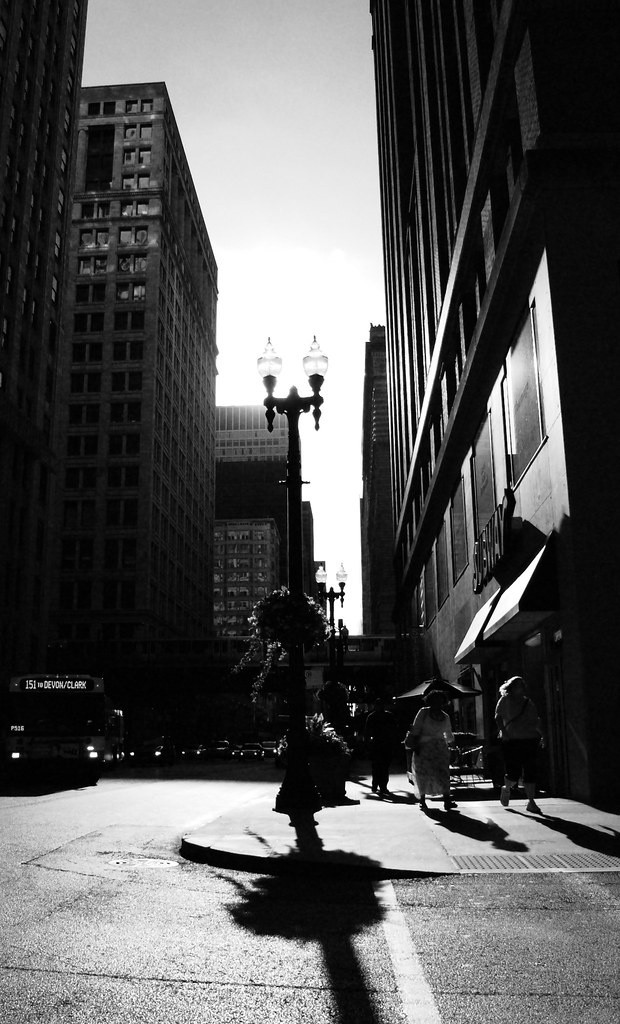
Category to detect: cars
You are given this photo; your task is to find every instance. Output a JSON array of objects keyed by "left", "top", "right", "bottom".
[{"left": 121, "top": 740, "right": 277, "bottom": 762}]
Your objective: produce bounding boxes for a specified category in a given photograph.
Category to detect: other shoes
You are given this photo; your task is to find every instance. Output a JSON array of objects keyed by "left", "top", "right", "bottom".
[
  {"left": 380, "top": 787, "right": 389, "bottom": 794},
  {"left": 500, "top": 785, "right": 510, "bottom": 806},
  {"left": 526, "top": 802, "right": 541, "bottom": 813},
  {"left": 420, "top": 800, "right": 427, "bottom": 810},
  {"left": 372, "top": 782, "right": 378, "bottom": 793},
  {"left": 444, "top": 801, "right": 457, "bottom": 810}
]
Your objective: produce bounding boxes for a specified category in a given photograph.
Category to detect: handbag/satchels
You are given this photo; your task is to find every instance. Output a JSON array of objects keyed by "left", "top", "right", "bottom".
[
  {"left": 405, "top": 733, "right": 422, "bottom": 755},
  {"left": 496, "top": 729, "right": 503, "bottom": 739}
]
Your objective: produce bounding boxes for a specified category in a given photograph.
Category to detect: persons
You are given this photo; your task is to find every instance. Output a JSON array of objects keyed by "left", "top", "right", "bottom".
[
  {"left": 405, "top": 692, "right": 463, "bottom": 810},
  {"left": 495, "top": 675, "right": 546, "bottom": 814},
  {"left": 363, "top": 698, "right": 399, "bottom": 799},
  {"left": 341, "top": 626, "right": 349, "bottom": 653}
]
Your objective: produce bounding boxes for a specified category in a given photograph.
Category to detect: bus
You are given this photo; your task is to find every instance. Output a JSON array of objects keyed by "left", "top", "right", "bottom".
[{"left": 7, "top": 675, "right": 105, "bottom": 784}]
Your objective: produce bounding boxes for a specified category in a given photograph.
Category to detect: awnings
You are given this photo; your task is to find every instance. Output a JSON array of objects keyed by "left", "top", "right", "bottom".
[
  {"left": 396, "top": 677, "right": 484, "bottom": 699},
  {"left": 453, "top": 531, "right": 566, "bottom": 664}
]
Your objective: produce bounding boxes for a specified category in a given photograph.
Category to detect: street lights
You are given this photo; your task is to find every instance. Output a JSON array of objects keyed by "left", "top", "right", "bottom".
[
  {"left": 256, "top": 336, "right": 330, "bottom": 813},
  {"left": 315, "top": 562, "right": 348, "bottom": 678}
]
